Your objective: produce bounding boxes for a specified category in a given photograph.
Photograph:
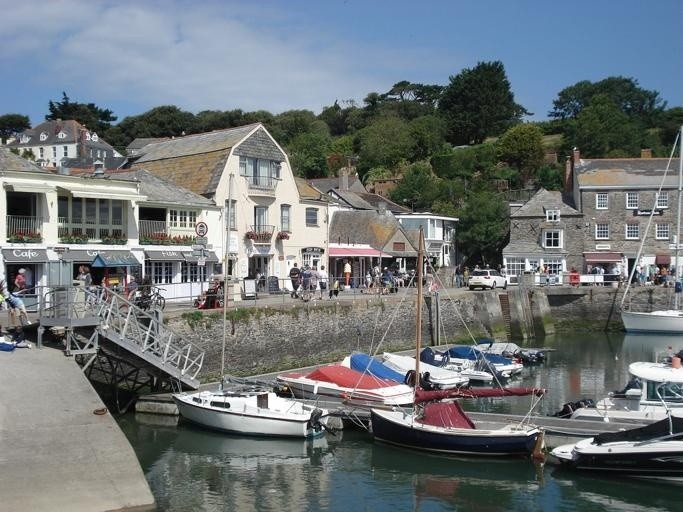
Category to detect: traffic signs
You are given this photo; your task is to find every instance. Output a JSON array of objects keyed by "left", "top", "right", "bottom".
[{"left": 195, "top": 222, "right": 208, "bottom": 236}]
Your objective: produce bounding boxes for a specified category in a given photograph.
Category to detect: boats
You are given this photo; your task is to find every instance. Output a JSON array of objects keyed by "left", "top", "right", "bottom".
[
  {"left": 443, "top": 346, "right": 523, "bottom": 379},
  {"left": 277, "top": 372, "right": 415, "bottom": 405},
  {"left": 420, "top": 346, "right": 495, "bottom": 381},
  {"left": 170, "top": 385, "right": 330, "bottom": 438},
  {"left": 551, "top": 424, "right": 683, "bottom": 481},
  {"left": 381, "top": 352, "right": 470, "bottom": 388},
  {"left": 341, "top": 350, "right": 442, "bottom": 391},
  {"left": 474, "top": 338, "right": 556, "bottom": 365}
]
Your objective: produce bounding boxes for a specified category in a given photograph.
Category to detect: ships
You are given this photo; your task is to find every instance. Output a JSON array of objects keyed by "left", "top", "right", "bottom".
[{"left": 567, "top": 347, "right": 683, "bottom": 422}]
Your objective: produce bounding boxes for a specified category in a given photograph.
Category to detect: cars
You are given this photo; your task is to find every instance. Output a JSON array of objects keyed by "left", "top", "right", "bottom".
[{"left": 468, "top": 269, "right": 508, "bottom": 291}]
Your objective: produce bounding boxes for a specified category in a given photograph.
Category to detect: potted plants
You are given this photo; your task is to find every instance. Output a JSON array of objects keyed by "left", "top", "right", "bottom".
[
  {"left": 8, "top": 231, "right": 42, "bottom": 244},
  {"left": 60, "top": 232, "right": 88, "bottom": 244},
  {"left": 246, "top": 231, "right": 272, "bottom": 241},
  {"left": 139, "top": 233, "right": 197, "bottom": 246},
  {"left": 277, "top": 232, "right": 289, "bottom": 240},
  {"left": 102, "top": 234, "right": 127, "bottom": 245}
]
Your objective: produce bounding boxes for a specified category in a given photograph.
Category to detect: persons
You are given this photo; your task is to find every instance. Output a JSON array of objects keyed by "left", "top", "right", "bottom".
[
  {"left": 14, "top": 267, "right": 30, "bottom": 305},
  {"left": 301, "top": 267, "right": 314, "bottom": 300},
  {"left": 75, "top": 265, "right": 92, "bottom": 306},
  {"left": 535, "top": 262, "right": 550, "bottom": 284},
  {"left": 318, "top": 265, "right": 330, "bottom": 299},
  {"left": 344, "top": 259, "right": 353, "bottom": 286},
  {"left": 463, "top": 266, "right": 469, "bottom": 289},
  {"left": 365, "top": 265, "right": 418, "bottom": 291},
  {"left": 474, "top": 264, "right": 481, "bottom": 270},
  {"left": 311, "top": 266, "right": 321, "bottom": 299},
  {"left": 500, "top": 264, "right": 506, "bottom": 278},
  {"left": 496, "top": 263, "right": 502, "bottom": 274},
  {"left": 288, "top": 262, "right": 303, "bottom": 299},
  {"left": 454, "top": 263, "right": 464, "bottom": 289},
  {"left": 485, "top": 263, "right": 490, "bottom": 270},
  {"left": 636, "top": 262, "right": 677, "bottom": 287},
  {"left": 0, "top": 262, "right": 33, "bottom": 327},
  {"left": 588, "top": 263, "right": 606, "bottom": 288}
]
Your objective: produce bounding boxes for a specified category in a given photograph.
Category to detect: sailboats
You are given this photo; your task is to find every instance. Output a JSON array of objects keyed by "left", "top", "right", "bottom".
[
  {"left": 370, "top": 225, "right": 549, "bottom": 457},
  {"left": 622, "top": 123, "right": 683, "bottom": 335}
]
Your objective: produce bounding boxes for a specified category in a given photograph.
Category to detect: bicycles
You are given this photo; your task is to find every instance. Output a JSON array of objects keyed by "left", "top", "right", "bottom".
[{"left": 150, "top": 285, "right": 167, "bottom": 311}]
